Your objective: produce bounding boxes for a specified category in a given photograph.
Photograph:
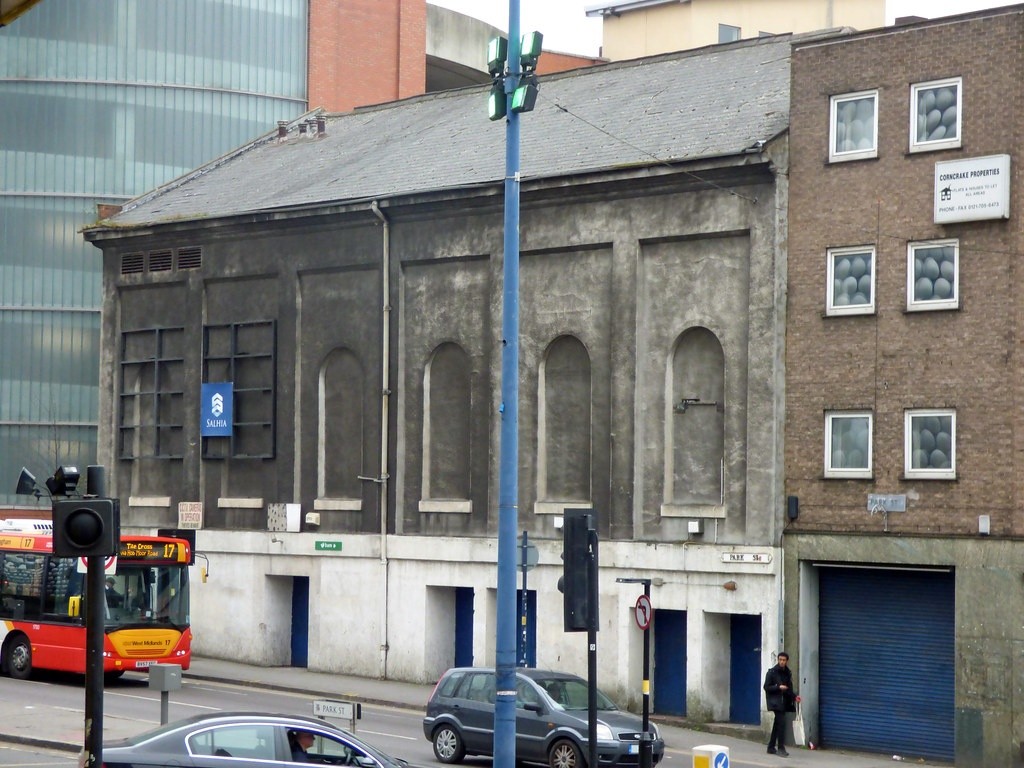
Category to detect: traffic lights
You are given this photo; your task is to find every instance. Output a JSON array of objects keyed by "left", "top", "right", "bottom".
[
  {"left": 557, "top": 508, "right": 599, "bottom": 633},
  {"left": 52, "top": 498, "right": 116, "bottom": 557}
]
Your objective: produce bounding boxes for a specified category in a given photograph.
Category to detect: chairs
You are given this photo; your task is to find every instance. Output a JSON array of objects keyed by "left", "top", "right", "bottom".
[
  {"left": 254, "top": 727, "right": 268, "bottom": 759},
  {"left": 549, "top": 683, "right": 563, "bottom": 704}
]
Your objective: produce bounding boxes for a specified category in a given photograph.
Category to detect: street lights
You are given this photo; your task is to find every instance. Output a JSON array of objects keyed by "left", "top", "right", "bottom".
[{"left": 486, "top": 32, "right": 539, "bottom": 768}]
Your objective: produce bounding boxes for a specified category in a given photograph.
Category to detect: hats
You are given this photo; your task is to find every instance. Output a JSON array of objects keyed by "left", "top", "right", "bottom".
[{"left": 106, "top": 577, "right": 116, "bottom": 584}]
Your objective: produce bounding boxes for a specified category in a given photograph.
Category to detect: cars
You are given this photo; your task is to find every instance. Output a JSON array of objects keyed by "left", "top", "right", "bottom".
[{"left": 79, "top": 711, "right": 422, "bottom": 768}]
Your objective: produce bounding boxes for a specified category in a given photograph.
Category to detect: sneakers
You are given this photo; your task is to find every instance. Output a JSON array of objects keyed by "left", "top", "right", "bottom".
[{"left": 767, "top": 747, "right": 789, "bottom": 757}]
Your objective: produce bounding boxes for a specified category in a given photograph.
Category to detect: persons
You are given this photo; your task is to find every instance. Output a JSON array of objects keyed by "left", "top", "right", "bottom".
[
  {"left": 292, "top": 731, "right": 315, "bottom": 762},
  {"left": 542, "top": 683, "right": 564, "bottom": 711},
  {"left": 764, "top": 652, "right": 801, "bottom": 757},
  {"left": 105, "top": 577, "right": 120, "bottom": 608},
  {"left": 131, "top": 591, "right": 147, "bottom": 612}
]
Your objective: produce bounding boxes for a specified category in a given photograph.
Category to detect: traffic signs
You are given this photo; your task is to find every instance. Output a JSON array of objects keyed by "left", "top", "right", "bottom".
[{"left": 313, "top": 699, "right": 353, "bottom": 721}]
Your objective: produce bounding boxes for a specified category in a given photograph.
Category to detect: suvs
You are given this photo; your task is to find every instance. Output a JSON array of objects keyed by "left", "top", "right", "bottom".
[{"left": 422, "top": 667, "right": 665, "bottom": 768}]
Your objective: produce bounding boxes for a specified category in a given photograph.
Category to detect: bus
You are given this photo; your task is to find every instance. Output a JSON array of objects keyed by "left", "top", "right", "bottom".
[{"left": 0, "top": 519, "right": 208, "bottom": 680}]
